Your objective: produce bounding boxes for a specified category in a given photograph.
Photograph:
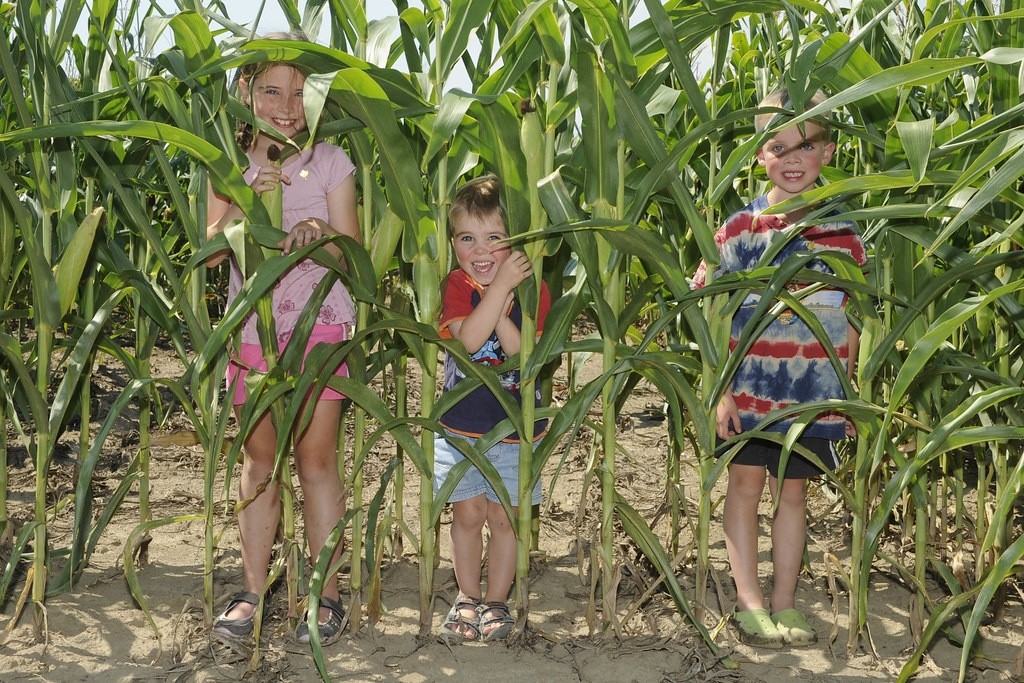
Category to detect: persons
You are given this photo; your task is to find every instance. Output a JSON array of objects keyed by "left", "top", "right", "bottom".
[
  {"left": 205, "top": 31, "right": 364, "bottom": 648},
  {"left": 429, "top": 173, "right": 554, "bottom": 645},
  {"left": 690, "top": 87, "right": 871, "bottom": 649}
]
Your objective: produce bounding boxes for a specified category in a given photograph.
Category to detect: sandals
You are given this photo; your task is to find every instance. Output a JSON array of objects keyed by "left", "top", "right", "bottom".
[
  {"left": 441, "top": 593, "right": 483, "bottom": 642},
  {"left": 768, "top": 603, "right": 818, "bottom": 647},
  {"left": 480, "top": 596, "right": 516, "bottom": 642},
  {"left": 731, "top": 603, "right": 785, "bottom": 649}
]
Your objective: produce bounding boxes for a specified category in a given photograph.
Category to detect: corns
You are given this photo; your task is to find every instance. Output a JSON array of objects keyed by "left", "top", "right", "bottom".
[
  {"left": 518, "top": 98, "right": 544, "bottom": 204},
  {"left": 54, "top": 205, "right": 104, "bottom": 316},
  {"left": 260, "top": 144, "right": 282, "bottom": 231},
  {"left": 369, "top": 203, "right": 404, "bottom": 282}
]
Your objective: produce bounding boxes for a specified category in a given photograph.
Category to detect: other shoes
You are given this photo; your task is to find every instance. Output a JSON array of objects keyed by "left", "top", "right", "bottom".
[
  {"left": 212, "top": 589, "right": 273, "bottom": 638},
  {"left": 296, "top": 592, "right": 344, "bottom": 647}
]
